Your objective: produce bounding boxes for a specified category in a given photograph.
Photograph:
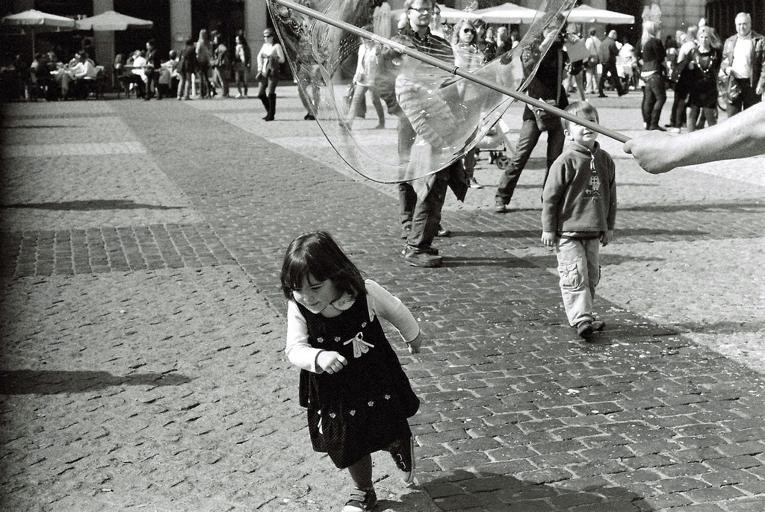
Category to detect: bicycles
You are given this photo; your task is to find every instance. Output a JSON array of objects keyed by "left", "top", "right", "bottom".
[{"left": 718, "top": 78, "right": 740, "bottom": 111}]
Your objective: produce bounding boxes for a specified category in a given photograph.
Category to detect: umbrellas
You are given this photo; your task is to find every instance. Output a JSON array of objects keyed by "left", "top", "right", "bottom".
[{"left": 4, "top": 10, "right": 77, "bottom": 32}]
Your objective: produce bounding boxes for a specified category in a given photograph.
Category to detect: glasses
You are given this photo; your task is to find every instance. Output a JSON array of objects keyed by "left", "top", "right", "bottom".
[{"left": 464, "top": 29, "right": 476, "bottom": 34}]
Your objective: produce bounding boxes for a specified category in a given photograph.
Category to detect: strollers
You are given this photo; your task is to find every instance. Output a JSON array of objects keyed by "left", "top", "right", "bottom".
[{"left": 464, "top": 122, "right": 510, "bottom": 170}]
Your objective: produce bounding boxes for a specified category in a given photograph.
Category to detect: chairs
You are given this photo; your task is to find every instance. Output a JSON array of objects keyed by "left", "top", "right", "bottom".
[{"left": 6, "top": 62, "right": 180, "bottom": 102}]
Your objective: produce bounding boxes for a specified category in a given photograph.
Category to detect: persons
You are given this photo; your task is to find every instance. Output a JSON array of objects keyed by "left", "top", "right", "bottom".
[
  {"left": 257, "top": 1, "right": 764, "bottom": 267},
  {"left": 280, "top": 229, "right": 422, "bottom": 512},
  {"left": 541, "top": 101, "right": 616, "bottom": 337},
  {"left": 29, "top": 25, "right": 251, "bottom": 101}
]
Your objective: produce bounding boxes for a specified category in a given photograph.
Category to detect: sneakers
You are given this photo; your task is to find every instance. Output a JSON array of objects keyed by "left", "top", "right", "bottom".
[
  {"left": 577, "top": 321, "right": 605, "bottom": 339},
  {"left": 342, "top": 486, "right": 377, "bottom": 512},
  {"left": 401, "top": 176, "right": 506, "bottom": 268},
  {"left": 391, "top": 433, "right": 415, "bottom": 484}
]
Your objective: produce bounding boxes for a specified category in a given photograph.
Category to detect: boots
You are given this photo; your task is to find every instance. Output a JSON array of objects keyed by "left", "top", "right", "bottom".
[{"left": 258, "top": 94, "right": 275, "bottom": 120}]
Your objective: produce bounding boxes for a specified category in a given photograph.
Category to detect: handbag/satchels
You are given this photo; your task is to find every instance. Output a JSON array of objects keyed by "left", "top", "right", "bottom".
[
  {"left": 716, "top": 74, "right": 743, "bottom": 105},
  {"left": 255, "top": 72, "right": 262, "bottom": 81},
  {"left": 343, "top": 96, "right": 366, "bottom": 118},
  {"left": 531, "top": 100, "right": 561, "bottom": 132},
  {"left": 449, "top": 161, "right": 470, "bottom": 202}
]
